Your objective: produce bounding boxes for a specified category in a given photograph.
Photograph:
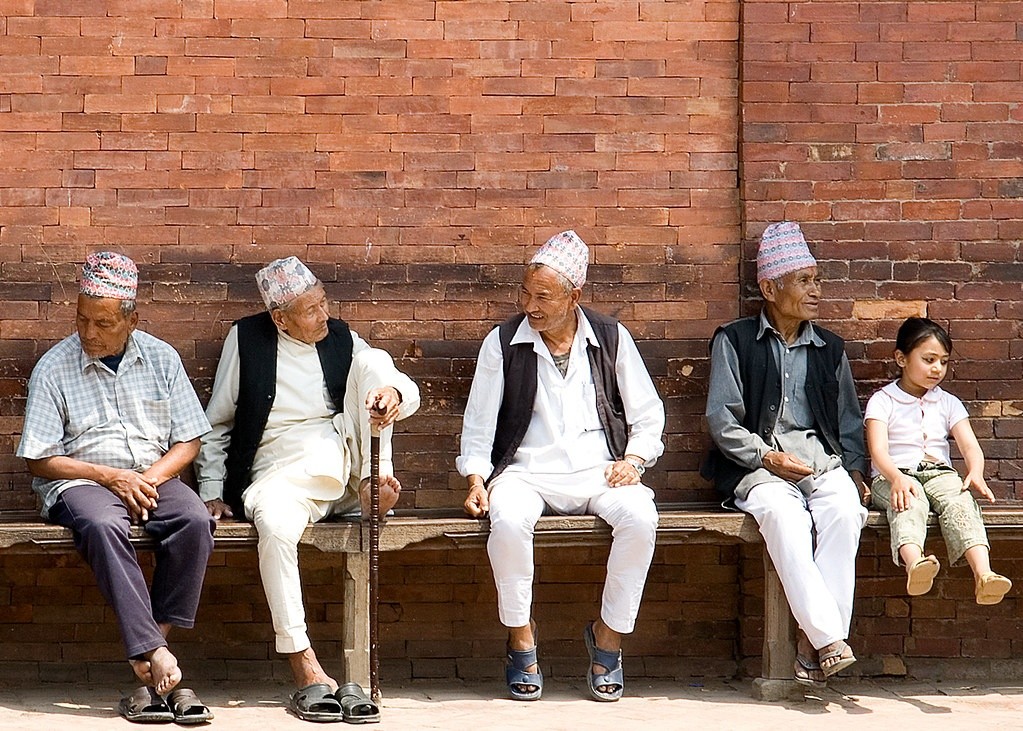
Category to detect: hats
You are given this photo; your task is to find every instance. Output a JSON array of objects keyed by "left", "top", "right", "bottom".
[
  {"left": 78, "top": 251, "right": 139, "bottom": 300},
  {"left": 527, "top": 229, "right": 591, "bottom": 291},
  {"left": 255, "top": 256, "right": 319, "bottom": 310},
  {"left": 756, "top": 220, "right": 817, "bottom": 282}
]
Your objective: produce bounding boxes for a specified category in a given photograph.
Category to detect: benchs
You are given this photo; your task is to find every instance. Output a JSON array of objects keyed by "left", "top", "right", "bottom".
[{"left": 0, "top": 499, "right": 1023, "bottom": 705}]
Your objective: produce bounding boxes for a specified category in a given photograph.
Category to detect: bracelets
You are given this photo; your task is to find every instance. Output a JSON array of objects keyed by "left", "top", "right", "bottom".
[
  {"left": 468, "top": 483, "right": 488, "bottom": 498},
  {"left": 625, "top": 458, "right": 646, "bottom": 478}
]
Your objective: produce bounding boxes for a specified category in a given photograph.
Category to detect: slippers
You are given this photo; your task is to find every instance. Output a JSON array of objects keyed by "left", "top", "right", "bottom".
[
  {"left": 906, "top": 556, "right": 941, "bottom": 596},
  {"left": 506, "top": 617, "right": 543, "bottom": 701},
  {"left": 974, "top": 571, "right": 1012, "bottom": 605},
  {"left": 167, "top": 688, "right": 215, "bottom": 724},
  {"left": 794, "top": 640, "right": 856, "bottom": 688},
  {"left": 584, "top": 622, "right": 624, "bottom": 702},
  {"left": 118, "top": 685, "right": 176, "bottom": 721},
  {"left": 288, "top": 682, "right": 344, "bottom": 722},
  {"left": 335, "top": 682, "right": 381, "bottom": 724}
]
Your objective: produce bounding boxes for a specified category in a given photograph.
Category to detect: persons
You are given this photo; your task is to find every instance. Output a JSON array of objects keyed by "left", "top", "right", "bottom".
[
  {"left": 704, "top": 219, "right": 873, "bottom": 689},
  {"left": 861, "top": 315, "right": 1013, "bottom": 607},
  {"left": 453, "top": 228, "right": 667, "bottom": 703},
  {"left": 14, "top": 250, "right": 216, "bottom": 696},
  {"left": 193, "top": 255, "right": 423, "bottom": 713}
]
